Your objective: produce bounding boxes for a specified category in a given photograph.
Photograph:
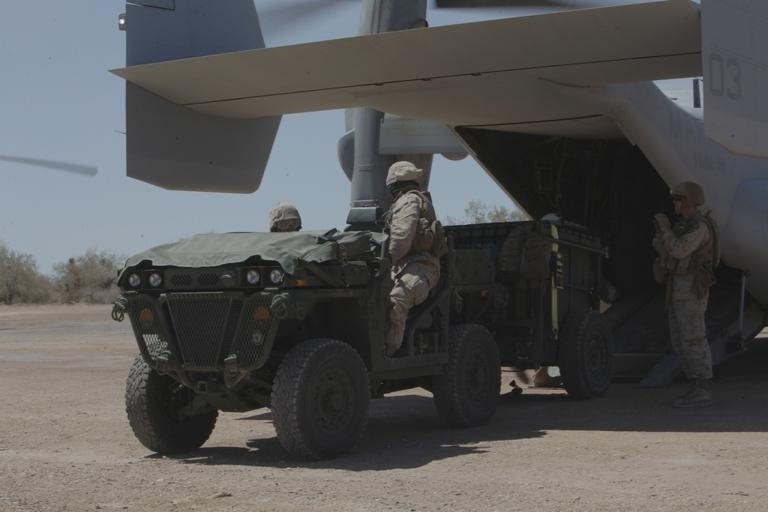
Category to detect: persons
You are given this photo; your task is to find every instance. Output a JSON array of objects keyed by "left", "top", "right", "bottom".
[
  {"left": 383, "top": 161, "right": 442, "bottom": 356},
  {"left": 512, "top": 366, "right": 562, "bottom": 387},
  {"left": 653, "top": 182, "right": 714, "bottom": 407}
]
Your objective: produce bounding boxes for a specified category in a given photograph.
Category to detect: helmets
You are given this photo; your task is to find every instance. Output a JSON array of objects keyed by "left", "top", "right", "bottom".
[
  {"left": 386, "top": 161, "right": 421, "bottom": 185},
  {"left": 670, "top": 182, "right": 704, "bottom": 205}
]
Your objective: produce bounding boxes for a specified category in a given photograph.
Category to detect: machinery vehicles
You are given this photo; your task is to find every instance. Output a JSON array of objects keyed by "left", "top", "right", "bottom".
[{"left": 111, "top": 219, "right": 614, "bottom": 461}]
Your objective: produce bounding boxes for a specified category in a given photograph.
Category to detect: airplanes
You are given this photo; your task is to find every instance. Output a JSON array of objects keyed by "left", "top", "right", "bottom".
[{"left": 109, "top": 0, "right": 768, "bottom": 388}]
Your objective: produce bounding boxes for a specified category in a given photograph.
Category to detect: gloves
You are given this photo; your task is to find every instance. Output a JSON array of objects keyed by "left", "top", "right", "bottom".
[{"left": 653, "top": 213, "right": 671, "bottom": 232}]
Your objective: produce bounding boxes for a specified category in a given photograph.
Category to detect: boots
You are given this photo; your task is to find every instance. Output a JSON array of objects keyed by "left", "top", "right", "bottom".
[{"left": 672, "top": 378, "right": 712, "bottom": 407}]
